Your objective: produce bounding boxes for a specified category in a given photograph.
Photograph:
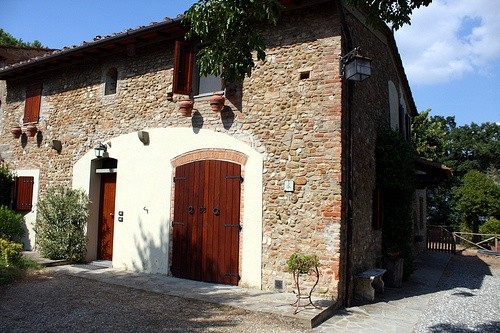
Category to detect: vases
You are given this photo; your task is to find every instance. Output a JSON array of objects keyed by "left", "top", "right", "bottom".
[
  {"left": 180, "top": 99, "right": 194, "bottom": 117},
  {"left": 26, "top": 123, "right": 37, "bottom": 138},
  {"left": 210, "top": 93, "right": 224, "bottom": 112},
  {"left": 11, "top": 127, "right": 21, "bottom": 139}
]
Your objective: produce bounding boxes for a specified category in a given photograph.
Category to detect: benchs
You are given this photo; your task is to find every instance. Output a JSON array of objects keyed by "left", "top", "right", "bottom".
[{"left": 354, "top": 267, "right": 388, "bottom": 302}]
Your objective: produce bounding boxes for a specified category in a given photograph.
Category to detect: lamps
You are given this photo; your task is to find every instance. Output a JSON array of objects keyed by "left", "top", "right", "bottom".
[
  {"left": 94, "top": 142, "right": 107, "bottom": 159},
  {"left": 339, "top": 46, "right": 371, "bottom": 82}
]
